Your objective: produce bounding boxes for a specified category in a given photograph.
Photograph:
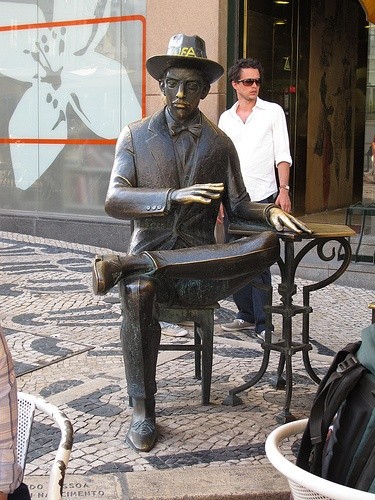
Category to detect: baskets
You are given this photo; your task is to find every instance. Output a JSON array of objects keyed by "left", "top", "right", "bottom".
[{"left": 265, "top": 418, "right": 375, "bottom": 500}]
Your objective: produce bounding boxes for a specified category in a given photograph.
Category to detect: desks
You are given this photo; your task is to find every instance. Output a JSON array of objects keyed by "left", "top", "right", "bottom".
[{"left": 227, "top": 219, "right": 356, "bottom": 424}]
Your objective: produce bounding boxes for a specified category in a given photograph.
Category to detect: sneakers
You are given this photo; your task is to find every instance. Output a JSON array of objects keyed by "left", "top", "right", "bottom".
[
  {"left": 257, "top": 330, "right": 281, "bottom": 343},
  {"left": 161, "top": 325, "right": 187, "bottom": 336},
  {"left": 221, "top": 318, "right": 256, "bottom": 331},
  {"left": 177, "top": 320, "right": 194, "bottom": 326}
]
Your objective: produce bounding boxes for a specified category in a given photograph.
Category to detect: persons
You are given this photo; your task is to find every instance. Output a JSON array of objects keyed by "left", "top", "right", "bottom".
[
  {"left": 371, "top": 135, "right": 375, "bottom": 170},
  {"left": 93, "top": 34, "right": 315, "bottom": 453},
  {"left": 218, "top": 58, "right": 292, "bottom": 344}
]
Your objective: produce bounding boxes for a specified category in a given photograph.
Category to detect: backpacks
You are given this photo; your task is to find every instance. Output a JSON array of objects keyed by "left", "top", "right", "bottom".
[{"left": 296, "top": 324, "right": 375, "bottom": 493}]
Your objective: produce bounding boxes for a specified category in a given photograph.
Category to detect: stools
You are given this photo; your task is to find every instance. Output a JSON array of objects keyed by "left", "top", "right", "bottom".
[{"left": 338, "top": 206, "right": 375, "bottom": 267}]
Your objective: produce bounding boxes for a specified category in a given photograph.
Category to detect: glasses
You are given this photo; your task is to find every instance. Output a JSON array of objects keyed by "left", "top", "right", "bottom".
[{"left": 238, "top": 79, "right": 261, "bottom": 86}]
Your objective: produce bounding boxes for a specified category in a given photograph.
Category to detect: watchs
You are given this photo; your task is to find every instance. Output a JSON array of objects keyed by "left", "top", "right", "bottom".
[{"left": 279, "top": 185, "right": 289, "bottom": 191}]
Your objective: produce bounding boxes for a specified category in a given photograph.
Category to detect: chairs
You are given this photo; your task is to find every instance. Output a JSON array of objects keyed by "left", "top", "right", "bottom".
[
  {"left": 153, "top": 302, "right": 221, "bottom": 407},
  {"left": 17, "top": 393, "right": 74, "bottom": 500},
  {"left": 265, "top": 419, "right": 375, "bottom": 500}
]
